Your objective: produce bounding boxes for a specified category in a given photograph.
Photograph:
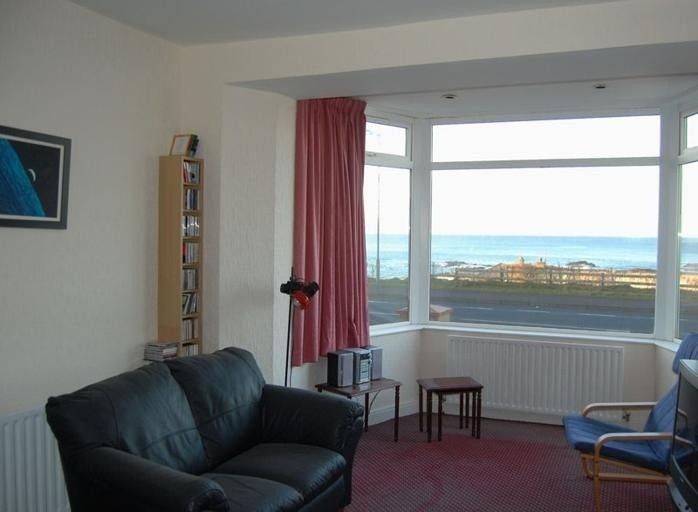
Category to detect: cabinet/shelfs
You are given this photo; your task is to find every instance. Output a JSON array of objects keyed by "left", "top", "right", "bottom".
[{"left": 157, "top": 155, "right": 204, "bottom": 359}]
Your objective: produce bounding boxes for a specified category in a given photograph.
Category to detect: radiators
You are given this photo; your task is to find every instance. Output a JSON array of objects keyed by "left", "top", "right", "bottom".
[{"left": 0, "top": 402, "right": 69, "bottom": 512}]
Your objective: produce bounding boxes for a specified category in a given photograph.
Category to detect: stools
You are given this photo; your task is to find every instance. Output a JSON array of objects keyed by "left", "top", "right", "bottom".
[{"left": 313, "top": 376, "right": 484, "bottom": 442}]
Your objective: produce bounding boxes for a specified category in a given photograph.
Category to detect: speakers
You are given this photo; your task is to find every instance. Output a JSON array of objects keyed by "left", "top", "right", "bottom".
[
  {"left": 327, "top": 350, "right": 354, "bottom": 387},
  {"left": 360, "top": 345, "right": 382, "bottom": 380}
]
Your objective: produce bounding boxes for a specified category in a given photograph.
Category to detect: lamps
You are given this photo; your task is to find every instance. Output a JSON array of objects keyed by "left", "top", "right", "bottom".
[{"left": 283, "top": 273, "right": 323, "bottom": 388}]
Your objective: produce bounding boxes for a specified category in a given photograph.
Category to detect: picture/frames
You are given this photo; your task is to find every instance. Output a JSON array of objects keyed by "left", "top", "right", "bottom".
[{"left": 0, "top": 124, "right": 72, "bottom": 230}]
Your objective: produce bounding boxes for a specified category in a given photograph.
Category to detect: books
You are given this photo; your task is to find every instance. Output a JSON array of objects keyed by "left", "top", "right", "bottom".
[{"left": 143, "top": 133, "right": 199, "bottom": 363}]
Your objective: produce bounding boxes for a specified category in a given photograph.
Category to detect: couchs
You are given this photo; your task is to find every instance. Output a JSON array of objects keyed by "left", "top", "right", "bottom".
[{"left": 45, "top": 346, "right": 364, "bottom": 512}]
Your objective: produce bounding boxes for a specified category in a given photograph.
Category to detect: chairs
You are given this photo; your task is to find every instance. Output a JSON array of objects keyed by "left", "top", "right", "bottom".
[{"left": 562, "top": 329, "right": 698, "bottom": 512}]
